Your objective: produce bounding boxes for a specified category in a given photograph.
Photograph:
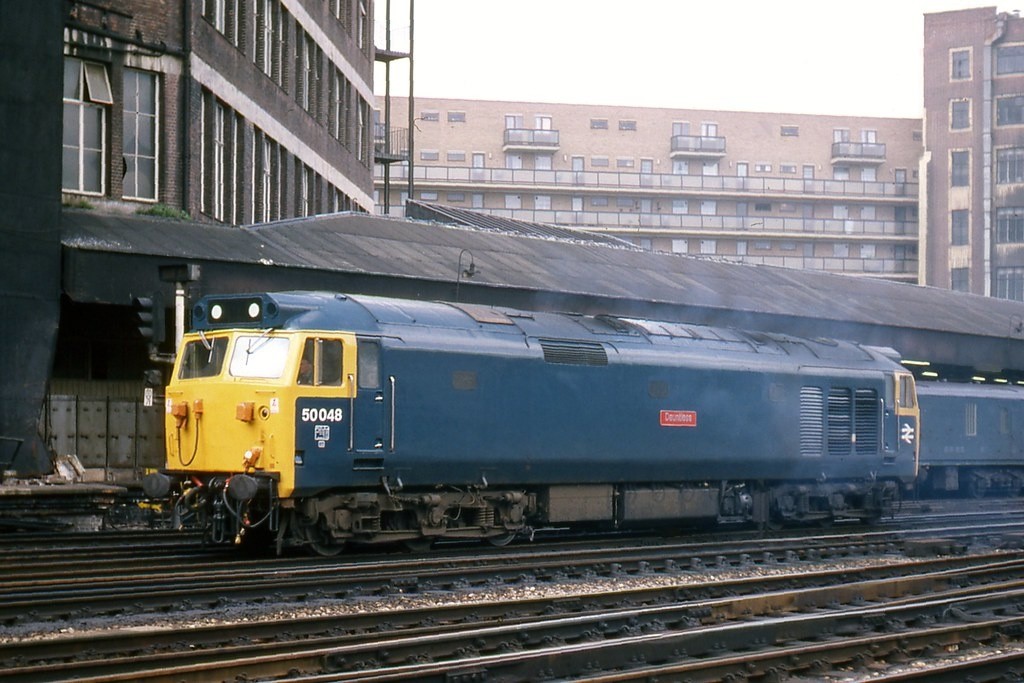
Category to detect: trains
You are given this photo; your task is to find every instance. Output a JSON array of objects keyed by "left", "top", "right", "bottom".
[{"left": 140, "top": 289, "right": 1024, "bottom": 559}]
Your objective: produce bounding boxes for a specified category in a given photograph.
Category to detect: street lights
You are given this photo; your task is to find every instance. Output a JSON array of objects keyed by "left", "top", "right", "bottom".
[{"left": 157, "top": 262, "right": 200, "bottom": 377}]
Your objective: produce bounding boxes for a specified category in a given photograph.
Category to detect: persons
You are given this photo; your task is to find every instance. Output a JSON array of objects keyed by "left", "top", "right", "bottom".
[{"left": 297, "top": 358, "right": 314, "bottom": 384}]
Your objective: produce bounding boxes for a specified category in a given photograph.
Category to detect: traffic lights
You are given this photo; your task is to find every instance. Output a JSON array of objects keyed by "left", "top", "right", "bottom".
[{"left": 133, "top": 291, "right": 165, "bottom": 345}]
